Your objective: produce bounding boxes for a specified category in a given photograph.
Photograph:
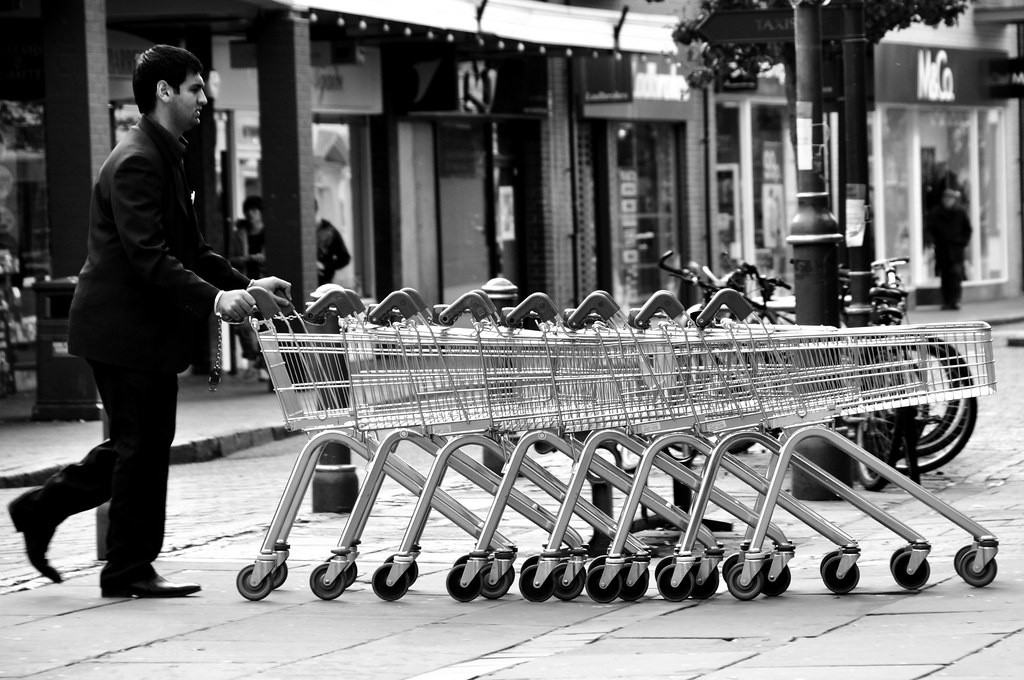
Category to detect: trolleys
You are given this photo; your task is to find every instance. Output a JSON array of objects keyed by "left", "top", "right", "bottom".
[{"left": 218, "top": 285, "right": 1001, "bottom": 602}]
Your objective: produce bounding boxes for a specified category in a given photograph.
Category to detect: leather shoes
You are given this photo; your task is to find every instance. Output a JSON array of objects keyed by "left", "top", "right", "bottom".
[
  {"left": 100, "top": 563, "right": 202, "bottom": 598},
  {"left": 8, "top": 492, "right": 64, "bottom": 584}
]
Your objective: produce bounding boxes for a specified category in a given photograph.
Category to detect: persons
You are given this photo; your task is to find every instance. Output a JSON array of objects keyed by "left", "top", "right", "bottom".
[
  {"left": 8, "top": 46, "right": 292, "bottom": 599},
  {"left": 230, "top": 196, "right": 270, "bottom": 381},
  {"left": 313, "top": 197, "right": 351, "bottom": 287},
  {"left": 934, "top": 184, "right": 974, "bottom": 310}
]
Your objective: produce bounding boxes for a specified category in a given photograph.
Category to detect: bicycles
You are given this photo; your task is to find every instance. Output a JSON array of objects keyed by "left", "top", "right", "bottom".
[{"left": 653, "top": 247, "right": 980, "bottom": 476}]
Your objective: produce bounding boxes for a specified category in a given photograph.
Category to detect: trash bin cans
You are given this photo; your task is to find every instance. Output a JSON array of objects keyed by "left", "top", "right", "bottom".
[{"left": 32, "top": 276, "right": 103, "bottom": 422}]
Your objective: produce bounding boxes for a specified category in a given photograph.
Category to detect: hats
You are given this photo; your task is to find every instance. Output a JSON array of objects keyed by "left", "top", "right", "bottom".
[{"left": 945, "top": 189, "right": 961, "bottom": 197}]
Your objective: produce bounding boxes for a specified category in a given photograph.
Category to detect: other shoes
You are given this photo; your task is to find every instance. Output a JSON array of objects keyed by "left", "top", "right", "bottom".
[
  {"left": 941, "top": 304, "right": 949, "bottom": 309},
  {"left": 951, "top": 304, "right": 959, "bottom": 310}
]
